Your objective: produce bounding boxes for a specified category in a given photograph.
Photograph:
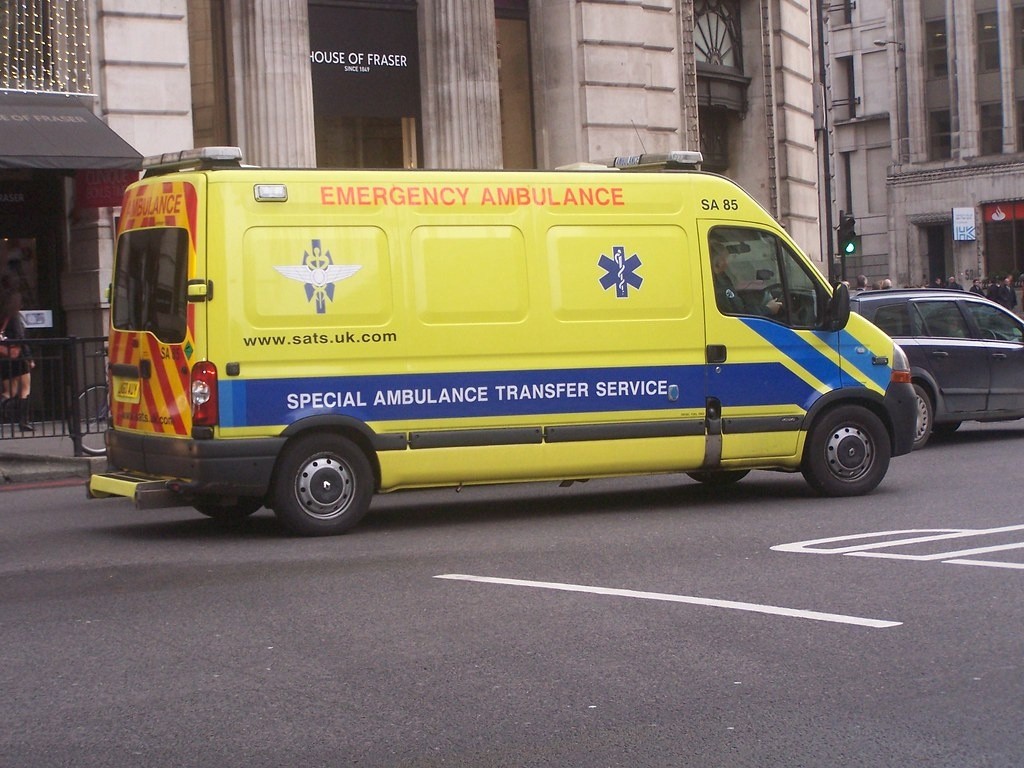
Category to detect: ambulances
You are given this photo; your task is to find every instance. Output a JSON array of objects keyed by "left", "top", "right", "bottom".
[{"left": 85, "top": 145, "right": 921, "bottom": 537}]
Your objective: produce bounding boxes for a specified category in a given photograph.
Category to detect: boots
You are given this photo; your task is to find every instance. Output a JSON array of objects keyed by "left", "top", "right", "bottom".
[
  {"left": 18, "top": 398, "right": 34, "bottom": 430},
  {"left": 2, "top": 395, "right": 9, "bottom": 408}
]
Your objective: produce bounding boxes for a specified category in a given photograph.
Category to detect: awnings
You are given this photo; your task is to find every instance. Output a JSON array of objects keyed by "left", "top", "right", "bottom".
[{"left": 0, "top": 88, "right": 145, "bottom": 176}]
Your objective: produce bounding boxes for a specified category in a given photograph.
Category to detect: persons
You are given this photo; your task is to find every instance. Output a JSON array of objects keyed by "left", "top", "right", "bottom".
[
  {"left": 0, "top": 289, "right": 36, "bottom": 433},
  {"left": 706, "top": 242, "right": 786, "bottom": 317},
  {"left": 831, "top": 271, "right": 1023, "bottom": 312}
]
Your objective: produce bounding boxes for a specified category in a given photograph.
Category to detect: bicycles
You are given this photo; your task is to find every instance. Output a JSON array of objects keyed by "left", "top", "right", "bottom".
[{"left": 79, "top": 349, "right": 108, "bottom": 457}]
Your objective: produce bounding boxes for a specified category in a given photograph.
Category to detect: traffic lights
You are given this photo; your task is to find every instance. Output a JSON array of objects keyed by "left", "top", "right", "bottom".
[{"left": 837, "top": 215, "right": 856, "bottom": 254}]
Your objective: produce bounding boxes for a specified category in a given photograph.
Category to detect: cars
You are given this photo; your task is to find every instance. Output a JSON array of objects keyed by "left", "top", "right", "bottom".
[{"left": 849, "top": 288, "right": 1024, "bottom": 451}]
